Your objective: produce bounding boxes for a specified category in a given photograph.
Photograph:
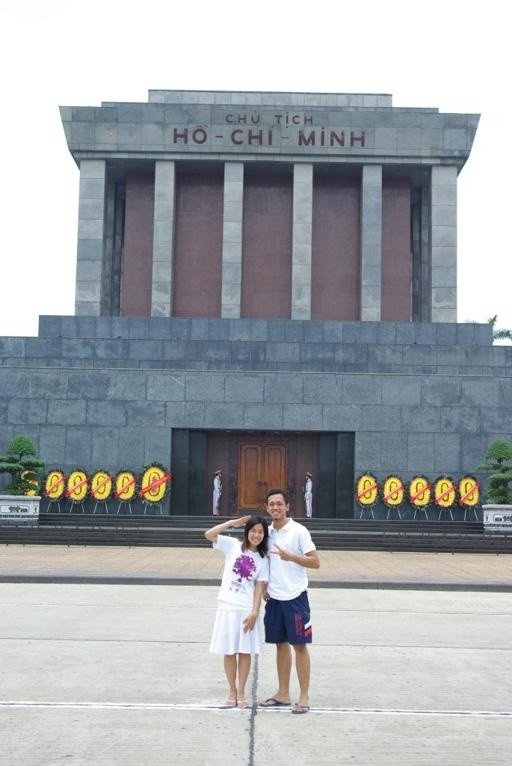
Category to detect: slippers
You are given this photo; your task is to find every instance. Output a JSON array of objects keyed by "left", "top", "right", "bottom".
[
  {"left": 292, "top": 702, "right": 310, "bottom": 714},
  {"left": 259, "top": 697, "right": 291, "bottom": 707},
  {"left": 226, "top": 699, "right": 249, "bottom": 708}
]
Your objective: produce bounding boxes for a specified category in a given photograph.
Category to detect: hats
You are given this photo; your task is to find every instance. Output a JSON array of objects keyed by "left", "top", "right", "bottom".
[
  {"left": 306, "top": 472, "right": 313, "bottom": 477},
  {"left": 214, "top": 469, "right": 223, "bottom": 474}
]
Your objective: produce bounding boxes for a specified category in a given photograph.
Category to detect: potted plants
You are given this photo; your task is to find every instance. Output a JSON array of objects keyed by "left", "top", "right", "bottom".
[
  {"left": 476, "top": 435, "right": 512, "bottom": 535},
  {"left": 0, "top": 434, "right": 41, "bottom": 530}
]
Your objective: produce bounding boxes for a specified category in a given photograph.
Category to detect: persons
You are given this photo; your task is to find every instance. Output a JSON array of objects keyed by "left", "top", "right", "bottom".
[
  {"left": 259, "top": 489, "right": 321, "bottom": 715},
  {"left": 304, "top": 472, "right": 313, "bottom": 518},
  {"left": 212, "top": 469, "right": 224, "bottom": 516},
  {"left": 204, "top": 516, "right": 271, "bottom": 710}
]
